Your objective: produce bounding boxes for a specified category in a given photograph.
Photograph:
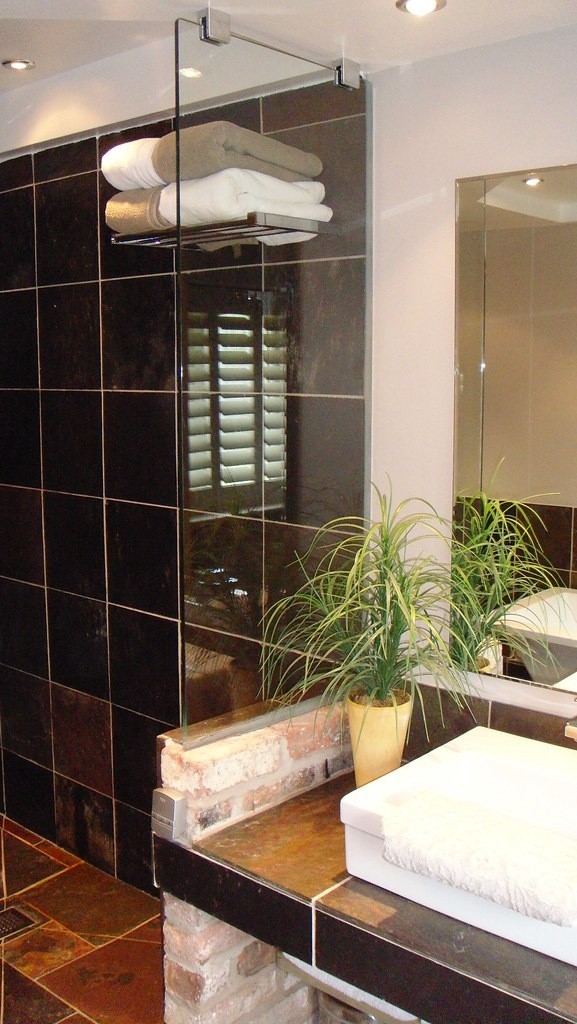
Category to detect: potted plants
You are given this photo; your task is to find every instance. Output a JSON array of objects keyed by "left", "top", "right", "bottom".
[
  {"left": 454, "top": 455, "right": 566, "bottom": 682},
  {"left": 257, "top": 469, "right": 490, "bottom": 788}
]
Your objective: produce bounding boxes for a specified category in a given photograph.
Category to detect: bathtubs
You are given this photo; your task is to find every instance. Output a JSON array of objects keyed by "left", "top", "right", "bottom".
[{"left": 479, "top": 586, "right": 577, "bottom": 685}]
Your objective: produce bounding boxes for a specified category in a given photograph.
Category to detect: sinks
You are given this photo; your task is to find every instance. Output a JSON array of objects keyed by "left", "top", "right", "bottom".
[{"left": 341, "top": 724, "right": 577, "bottom": 969}]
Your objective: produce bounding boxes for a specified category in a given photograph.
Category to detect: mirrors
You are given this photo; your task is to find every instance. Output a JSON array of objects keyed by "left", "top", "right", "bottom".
[{"left": 448, "top": 164, "right": 577, "bottom": 695}]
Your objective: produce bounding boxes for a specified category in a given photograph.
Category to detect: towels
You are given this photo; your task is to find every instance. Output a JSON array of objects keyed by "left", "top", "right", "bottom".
[
  {"left": 101, "top": 137, "right": 165, "bottom": 190},
  {"left": 160, "top": 168, "right": 335, "bottom": 246},
  {"left": 105, "top": 188, "right": 259, "bottom": 251},
  {"left": 153, "top": 121, "right": 324, "bottom": 184}
]
